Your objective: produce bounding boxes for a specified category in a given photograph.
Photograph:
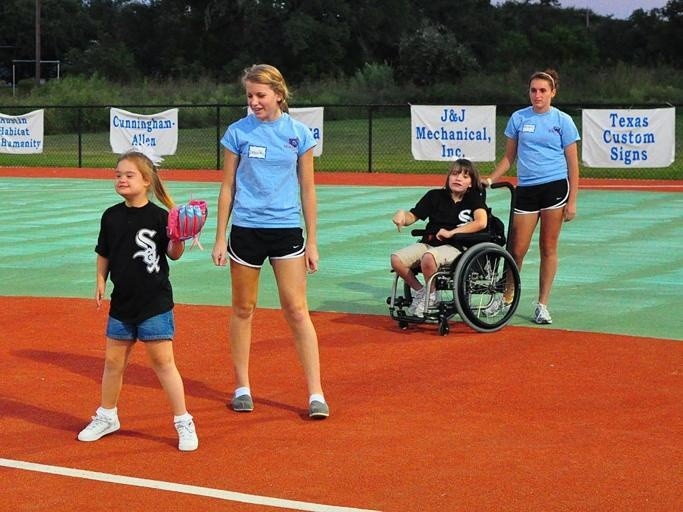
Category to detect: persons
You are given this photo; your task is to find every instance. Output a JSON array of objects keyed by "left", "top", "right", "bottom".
[
  {"left": 77, "top": 152, "right": 208, "bottom": 452},
  {"left": 391, "top": 159, "right": 487, "bottom": 318},
  {"left": 480, "top": 69, "right": 580, "bottom": 324},
  {"left": 212, "top": 64, "right": 329, "bottom": 418}
]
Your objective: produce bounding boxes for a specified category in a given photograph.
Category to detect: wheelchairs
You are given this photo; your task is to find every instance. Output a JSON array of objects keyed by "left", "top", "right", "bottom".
[{"left": 384, "top": 180, "right": 521, "bottom": 334}]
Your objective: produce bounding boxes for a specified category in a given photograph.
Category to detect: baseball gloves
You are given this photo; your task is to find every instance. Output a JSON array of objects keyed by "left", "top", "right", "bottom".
[{"left": 167, "top": 199, "right": 208, "bottom": 243}]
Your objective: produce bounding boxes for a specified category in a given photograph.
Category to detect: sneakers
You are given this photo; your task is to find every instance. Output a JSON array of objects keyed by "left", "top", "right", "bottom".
[
  {"left": 484, "top": 298, "right": 514, "bottom": 317},
  {"left": 309, "top": 401, "right": 329, "bottom": 419},
  {"left": 231, "top": 394, "right": 253, "bottom": 411},
  {"left": 77, "top": 410, "right": 120, "bottom": 442},
  {"left": 532, "top": 301, "right": 552, "bottom": 323},
  {"left": 174, "top": 415, "right": 198, "bottom": 451},
  {"left": 407, "top": 289, "right": 438, "bottom": 319}
]
